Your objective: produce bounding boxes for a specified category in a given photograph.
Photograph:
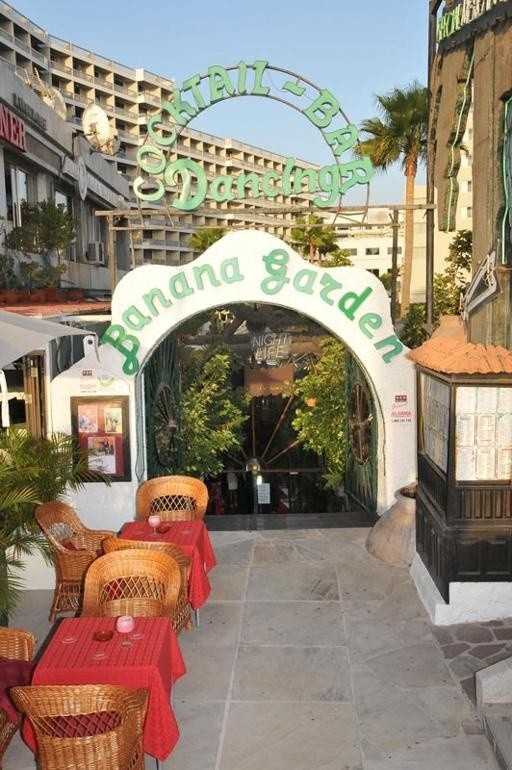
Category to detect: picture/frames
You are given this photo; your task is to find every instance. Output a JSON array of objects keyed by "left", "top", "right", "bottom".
[{"left": 70, "top": 394, "right": 131, "bottom": 483}]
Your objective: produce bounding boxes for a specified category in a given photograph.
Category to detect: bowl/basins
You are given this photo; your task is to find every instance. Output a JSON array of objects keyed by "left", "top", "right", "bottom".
[
  {"left": 157, "top": 526, "right": 170, "bottom": 534},
  {"left": 93, "top": 629, "right": 114, "bottom": 641}
]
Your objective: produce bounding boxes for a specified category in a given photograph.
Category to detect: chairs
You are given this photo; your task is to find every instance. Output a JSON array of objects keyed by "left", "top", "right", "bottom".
[
  {"left": 135, "top": 474, "right": 210, "bottom": 521},
  {"left": 10, "top": 683, "right": 151, "bottom": 770},
  {"left": 102, "top": 537, "right": 191, "bottom": 639},
  {"left": 0, "top": 626, "right": 36, "bottom": 770},
  {"left": 79, "top": 547, "right": 181, "bottom": 617},
  {"left": 35, "top": 500, "right": 115, "bottom": 623}
]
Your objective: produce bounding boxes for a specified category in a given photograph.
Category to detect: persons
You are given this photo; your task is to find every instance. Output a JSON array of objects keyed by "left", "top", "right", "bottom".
[{"left": 103, "top": 438, "right": 110, "bottom": 455}]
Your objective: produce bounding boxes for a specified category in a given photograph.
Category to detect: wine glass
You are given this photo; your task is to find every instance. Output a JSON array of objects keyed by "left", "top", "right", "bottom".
[
  {"left": 116, "top": 615, "right": 135, "bottom": 648},
  {"left": 148, "top": 516, "right": 161, "bottom": 537}
]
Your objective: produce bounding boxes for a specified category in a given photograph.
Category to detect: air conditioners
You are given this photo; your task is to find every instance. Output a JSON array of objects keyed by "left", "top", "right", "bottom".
[{"left": 88, "top": 241, "right": 105, "bottom": 265}]
